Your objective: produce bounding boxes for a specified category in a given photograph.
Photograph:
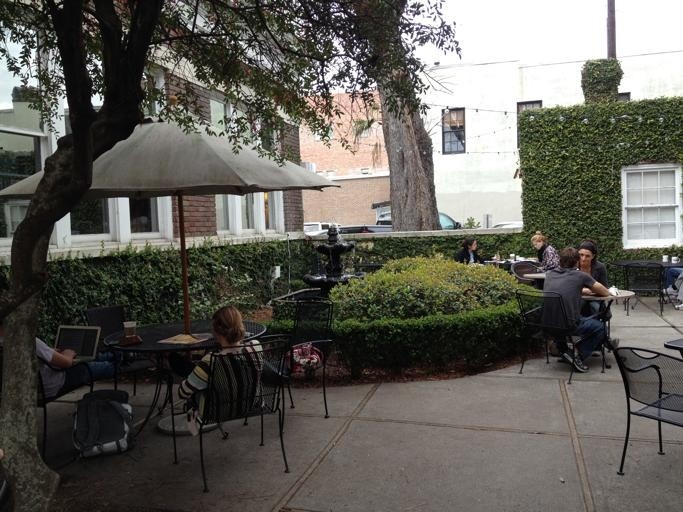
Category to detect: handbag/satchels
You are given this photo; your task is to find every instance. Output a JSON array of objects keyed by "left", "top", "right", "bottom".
[{"left": 285, "top": 342, "right": 324, "bottom": 378}]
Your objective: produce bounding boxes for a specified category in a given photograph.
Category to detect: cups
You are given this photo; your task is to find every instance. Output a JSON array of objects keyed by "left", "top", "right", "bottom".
[
  {"left": 123, "top": 321, "right": 136, "bottom": 338},
  {"left": 494, "top": 253, "right": 525, "bottom": 261},
  {"left": 662, "top": 255, "right": 680, "bottom": 264}
]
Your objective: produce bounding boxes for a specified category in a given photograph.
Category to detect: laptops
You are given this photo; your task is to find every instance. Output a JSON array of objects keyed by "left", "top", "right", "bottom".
[{"left": 52, "top": 325, "right": 101, "bottom": 362}]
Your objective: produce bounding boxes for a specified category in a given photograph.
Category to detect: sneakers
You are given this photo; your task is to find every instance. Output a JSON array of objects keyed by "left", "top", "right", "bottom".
[
  {"left": 563, "top": 348, "right": 589, "bottom": 373},
  {"left": 662, "top": 285, "right": 678, "bottom": 296},
  {"left": 606, "top": 337, "right": 620, "bottom": 353}
]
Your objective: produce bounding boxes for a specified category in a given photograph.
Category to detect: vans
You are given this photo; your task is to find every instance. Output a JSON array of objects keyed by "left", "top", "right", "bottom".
[{"left": 303, "top": 221, "right": 341, "bottom": 231}]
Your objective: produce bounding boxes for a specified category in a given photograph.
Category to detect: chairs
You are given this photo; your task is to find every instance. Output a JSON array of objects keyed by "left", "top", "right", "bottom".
[
  {"left": 76, "top": 303, "right": 158, "bottom": 397},
  {"left": 483, "top": 254, "right": 682, "bottom": 384},
  {"left": 185, "top": 339, "right": 290, "bottom": 495},
  {"left": 32, "top": 353, "right": 95, "bottom": 462},
  {"left": 613, "top": 346, "right": 682, "bottom": 476},
  {"left": 259, "top": 296, "right": 334, "bottom": 420}
]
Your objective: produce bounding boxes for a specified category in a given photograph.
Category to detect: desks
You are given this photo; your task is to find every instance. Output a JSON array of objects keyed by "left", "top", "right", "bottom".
[{"left": 103, "top": 318, "right": 270, "bottom": 465}]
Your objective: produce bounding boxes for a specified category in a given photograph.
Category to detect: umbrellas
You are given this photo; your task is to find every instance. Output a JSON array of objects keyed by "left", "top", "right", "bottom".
[{"left": 0, "top": 96, "right": 345, "bottom": 334}]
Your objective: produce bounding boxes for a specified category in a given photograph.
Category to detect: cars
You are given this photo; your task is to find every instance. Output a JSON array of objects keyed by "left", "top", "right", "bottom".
[
  {"left": 304, "top": 229, "right": 341, "bottom": 236},
  {"left": 490, "top": 221, "right": 523, "bottom": 229}
]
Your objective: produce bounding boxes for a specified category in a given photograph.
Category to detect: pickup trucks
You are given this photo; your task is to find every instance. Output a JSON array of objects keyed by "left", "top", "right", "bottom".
[{"left": 326, "top": 208, "right": 462, "bottom": 234}]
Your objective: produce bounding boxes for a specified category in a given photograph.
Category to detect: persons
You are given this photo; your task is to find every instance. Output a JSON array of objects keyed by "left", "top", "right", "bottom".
[
  {"left": 453, "top": 237, "right": 483, "bottom": 264},
  {"left": 540, "top": 244, "right": 609, "bottom": 374},
  {"left": 176, "top": 305, "right": 265, "bottom": 423},
  {"left": 661, "top": 266, "right": 682, "bottom": 310},
  {"left": 35, "top": 333, "right": 128, "bottom": 404},
  {"left": 530, "top": 231, "right": 559, "bottom": 273},
  {"left": 575, "top": 239, "right": 619, "bottom": 357}
]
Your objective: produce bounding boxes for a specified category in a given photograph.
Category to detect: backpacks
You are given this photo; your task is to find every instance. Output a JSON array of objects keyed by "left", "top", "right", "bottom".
[{"left": 71, "top": 389, "right": 135, "bottom": 458}]
